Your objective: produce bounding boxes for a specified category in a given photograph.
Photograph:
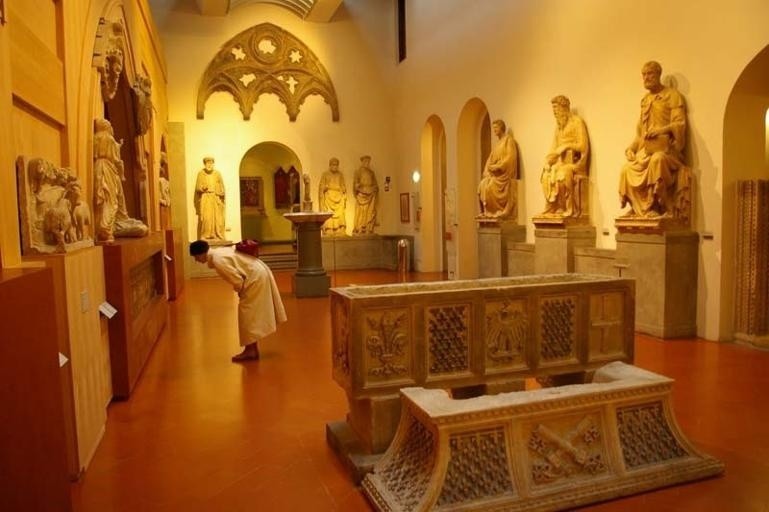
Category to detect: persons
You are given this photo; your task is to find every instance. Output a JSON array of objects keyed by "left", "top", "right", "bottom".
[
  {"left": 190, "top": 239, "right": 287, "bottom": 362},
  {"left": 110, "top": 125, "right": 141, "bottom": 228},
  {"left": 352, "top": 155, "right": 378, "bottom": 235},
  {"left": 619, "top": 59, "right": 688, "bottom": 216},
  {"left": 319, "top": 156, "right": 349, "bottom": 238},
  {"left": 475, "top": 119, "right": 519, "bottom": 220},
  {"left": 159, "top": 152, "right": 171, "bottom": 231},
  {"left": 94, "top": 117, "right": 119, "bottom": 243},
  {"left": 540, "top": 95, "right": 590, "bottom": 218},
  {"left": 195, "top": 156, "right": 225, "bottom": 241}
]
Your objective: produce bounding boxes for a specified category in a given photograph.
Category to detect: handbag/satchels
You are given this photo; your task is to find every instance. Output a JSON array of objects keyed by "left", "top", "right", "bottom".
[{"left": 235, "top": 238, "right": 261, "bottom": 259}]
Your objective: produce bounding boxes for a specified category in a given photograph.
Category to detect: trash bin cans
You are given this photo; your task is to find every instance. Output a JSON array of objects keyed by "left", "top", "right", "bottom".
[{"left": 397, "top": 239, "right": 411, "bottom": 274}]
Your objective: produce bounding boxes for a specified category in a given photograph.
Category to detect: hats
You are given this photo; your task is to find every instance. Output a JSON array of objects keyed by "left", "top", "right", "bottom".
[{"left": 189, "top": 239, "right": 210, "bottom": 256}]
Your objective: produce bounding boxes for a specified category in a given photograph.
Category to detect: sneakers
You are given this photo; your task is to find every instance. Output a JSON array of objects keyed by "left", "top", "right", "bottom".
[{"left": 231, "top": 350, "right": 260, "bottom": 362}]
